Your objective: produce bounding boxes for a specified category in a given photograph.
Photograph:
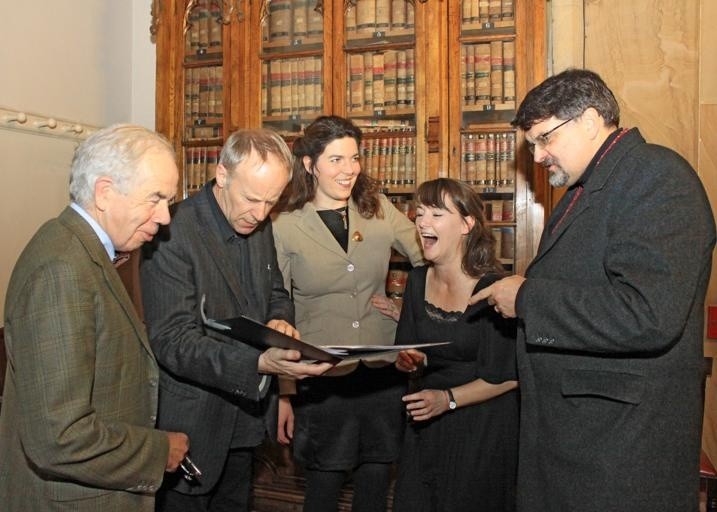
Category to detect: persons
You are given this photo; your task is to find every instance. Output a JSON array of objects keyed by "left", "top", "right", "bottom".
[
  {"left": 2, "top": 122, "right": 192, "bottom": 510},
  {"left": 392, "top": 176, "right": 519, "bottom": 511},
  {"left": 462, "top": 67, "right": 715, "bottom": 511},
  {"left": 265, "top": 114, "right": 432, "bottom": 510},
  {"left": 137, "top": 124, "right": 337, "bottom": 512}
]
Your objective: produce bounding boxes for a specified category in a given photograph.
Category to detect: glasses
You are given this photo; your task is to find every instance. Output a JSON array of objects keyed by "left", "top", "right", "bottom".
[
  {"left": 528, "top": 109, "right": 586, "bottom": 157},
  {"left": 178, "top": 454, "right": 203, "bottom": 482}
]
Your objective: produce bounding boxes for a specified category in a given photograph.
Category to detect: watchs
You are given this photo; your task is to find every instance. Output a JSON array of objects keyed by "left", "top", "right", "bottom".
[{"left": 445, "top": 387, "right": 457, "bottom": 413}]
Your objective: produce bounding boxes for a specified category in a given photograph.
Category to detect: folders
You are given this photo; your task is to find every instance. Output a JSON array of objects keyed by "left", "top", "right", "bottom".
[{"left": 219, "top": 314, "right": 449, "bottom": 375}]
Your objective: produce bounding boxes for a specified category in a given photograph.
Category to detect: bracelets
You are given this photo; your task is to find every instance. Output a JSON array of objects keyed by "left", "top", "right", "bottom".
[{"left": 441, "top": 389, "right": 449, "bottom": 412}]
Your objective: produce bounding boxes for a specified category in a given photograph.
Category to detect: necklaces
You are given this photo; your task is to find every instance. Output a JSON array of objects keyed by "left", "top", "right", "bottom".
[{"left": 310, "top": 194, "right": 350, "bottom": 234}]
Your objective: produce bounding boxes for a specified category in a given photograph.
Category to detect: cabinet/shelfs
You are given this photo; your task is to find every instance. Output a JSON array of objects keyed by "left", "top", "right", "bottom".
[{"left": 149, "top": 0, "right": 535, "bottom": 512}]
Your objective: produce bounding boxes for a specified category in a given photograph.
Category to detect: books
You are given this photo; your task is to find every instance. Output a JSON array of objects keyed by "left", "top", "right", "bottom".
[
  {"left": 461, "top": 1, "right": 516, "bottom": 141},
  {"left": 259, "top": 0, "right": 325, "bottom": 115},
  {"left": 342, "top": 0, "right": 418, "bottom": 141},
  {"left": 180, "top": 142, "right": 222, "bottom": 203},
  {"left": 358, "top": 136, "right": 417, "bottom": 310},
  {"left": 458, "top": 131, "right": 519, "bottom": 264},
  {"left": 182, "top": 1, "right": 224, "bottom": 141}
]
